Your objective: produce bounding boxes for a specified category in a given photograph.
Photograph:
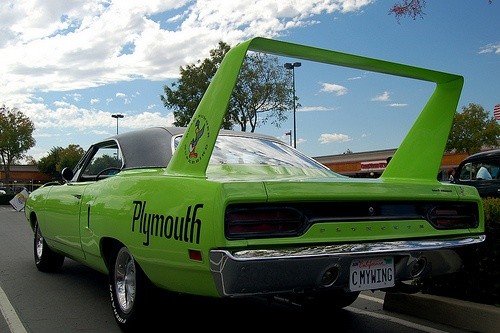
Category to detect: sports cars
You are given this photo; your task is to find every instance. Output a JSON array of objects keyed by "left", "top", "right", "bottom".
[{"left": 25, "top": 37, "right": 486, "bottom": 328}]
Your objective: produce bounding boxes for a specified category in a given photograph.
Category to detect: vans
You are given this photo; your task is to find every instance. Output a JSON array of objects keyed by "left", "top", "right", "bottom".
[{"left": 448, "top": 150, "right": 500, "bottom": 198}]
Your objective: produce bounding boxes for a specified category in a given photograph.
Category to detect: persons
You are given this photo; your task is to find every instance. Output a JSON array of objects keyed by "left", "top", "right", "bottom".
[
  {"left": 448, "top": 168, "right": 455, "bottom": 182},
  {"left": 476, "top": 163, "right": 493, "bottom": 180}
]
